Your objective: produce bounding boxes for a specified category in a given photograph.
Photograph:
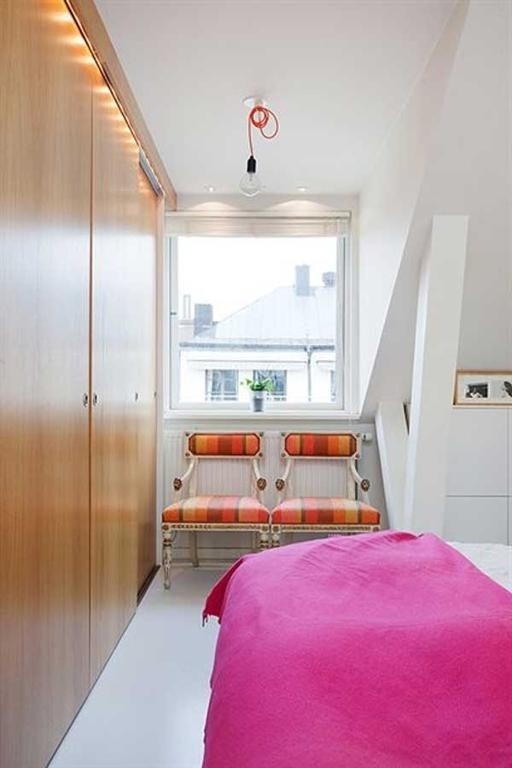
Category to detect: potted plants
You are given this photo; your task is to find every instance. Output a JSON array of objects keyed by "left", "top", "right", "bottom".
[{"left": 244, "top": 377, "right": 273, "bottom": 412}]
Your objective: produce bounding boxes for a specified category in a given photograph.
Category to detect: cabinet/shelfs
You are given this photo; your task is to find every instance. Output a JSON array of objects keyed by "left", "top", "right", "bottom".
[{"left": 2, "top": 4, "right": 175, "bottom": 761}]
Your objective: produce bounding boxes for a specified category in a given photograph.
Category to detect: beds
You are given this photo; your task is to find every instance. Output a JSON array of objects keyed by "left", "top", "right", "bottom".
[{"left": 201, "top": 535, "right": 512, "bottom": 767}]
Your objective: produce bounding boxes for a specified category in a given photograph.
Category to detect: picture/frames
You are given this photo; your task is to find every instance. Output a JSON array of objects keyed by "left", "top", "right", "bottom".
[{"left": 453, "top": 369, "right": 511, "bottom": 407}]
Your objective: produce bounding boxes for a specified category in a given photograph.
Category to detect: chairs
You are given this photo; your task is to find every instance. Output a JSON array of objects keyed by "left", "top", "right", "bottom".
[{"left": 159, "top": 427, "right": 382, "bottom": 594}]
